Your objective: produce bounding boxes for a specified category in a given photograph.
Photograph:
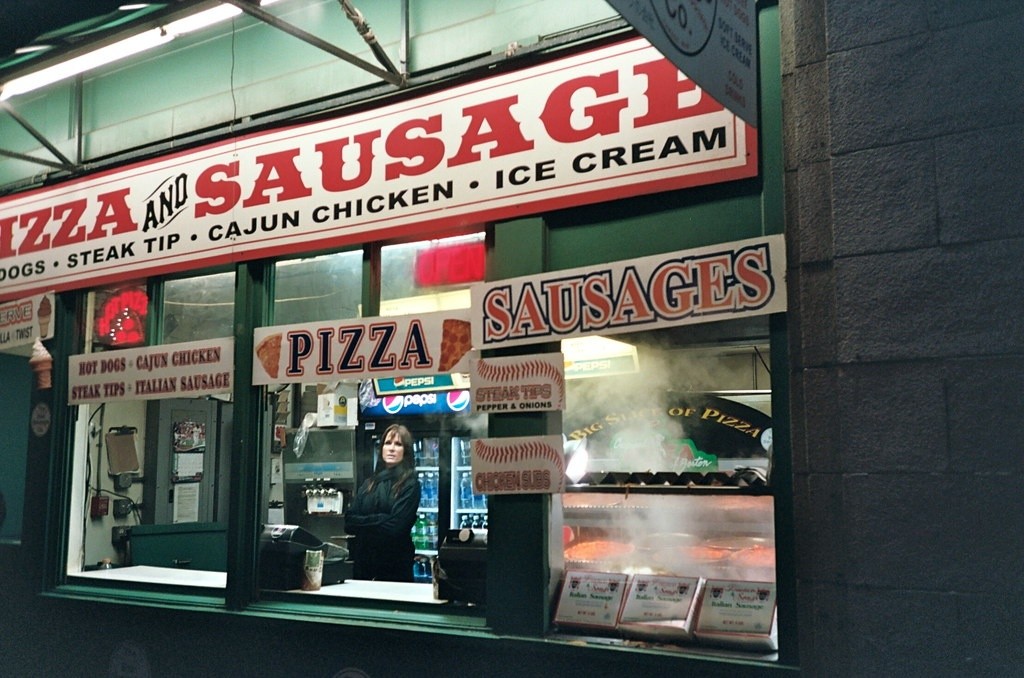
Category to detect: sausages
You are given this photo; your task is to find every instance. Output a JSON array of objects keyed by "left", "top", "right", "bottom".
[{"left": 29, "top": 337, "right": 52, "bottom": 390}]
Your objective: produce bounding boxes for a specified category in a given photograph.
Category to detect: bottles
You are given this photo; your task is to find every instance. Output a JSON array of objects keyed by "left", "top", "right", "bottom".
[
  {"left": 377, "top": 438, "right": 488, "bottom": 583},
  {"left": 101, "top": 559, "right": 112, "bottom": 569}
]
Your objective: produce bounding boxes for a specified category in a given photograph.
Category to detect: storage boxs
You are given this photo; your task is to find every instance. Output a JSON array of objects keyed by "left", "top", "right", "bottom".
[{"left": 316, "top": 382, "right": 358, "bottom": 428}]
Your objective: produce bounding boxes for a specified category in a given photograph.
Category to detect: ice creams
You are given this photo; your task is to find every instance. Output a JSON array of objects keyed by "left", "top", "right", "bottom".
[{"left": 37, "top": 295, "right": 51, "bottom": 338}]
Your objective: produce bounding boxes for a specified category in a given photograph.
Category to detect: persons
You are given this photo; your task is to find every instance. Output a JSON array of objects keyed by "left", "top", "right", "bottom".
[
  {"left": 174, "top": 423, "right": 204, "bottom": 449},
  {"left": 344, "top": 424, "right": 420, "bottom": 583}
]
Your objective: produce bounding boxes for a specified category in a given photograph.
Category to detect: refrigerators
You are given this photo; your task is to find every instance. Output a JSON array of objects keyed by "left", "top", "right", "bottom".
[{"left": 359, "top": 373, "right": 488, "bottom": 579}]
